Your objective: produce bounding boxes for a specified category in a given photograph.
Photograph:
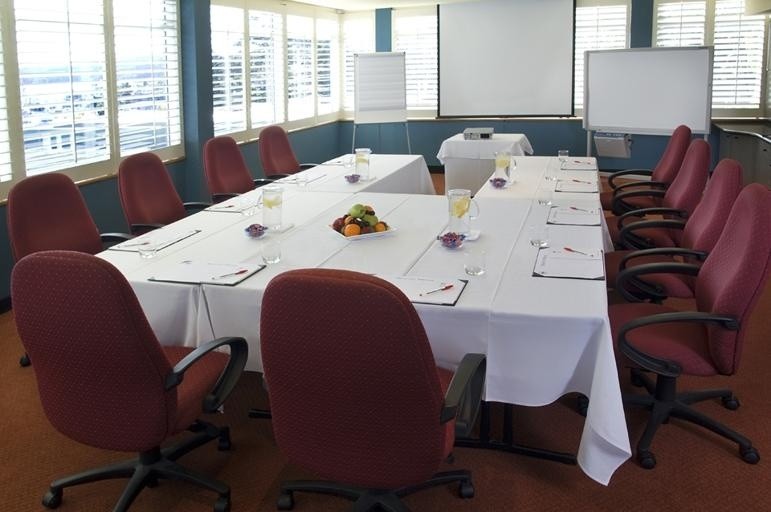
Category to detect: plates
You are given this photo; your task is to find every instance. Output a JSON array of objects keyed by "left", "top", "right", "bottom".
[{"left": 326, "top": 222, "right": 401, "bottom": 241}]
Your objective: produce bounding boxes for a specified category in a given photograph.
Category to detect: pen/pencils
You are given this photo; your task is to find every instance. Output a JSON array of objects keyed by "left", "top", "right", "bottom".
[
  {"left": 574, "top": 161, "right": 590, "bottom": 164},
  {"left": 216, "top": 205, "right": 234, "bottom": 208},
  {"left": 126, "top": 243, "right": 150, "bottom": 246},
  {"left": 573, "top": 180, "right": 591, "bottom": 184},
  {"left": 570, "top": 206, "right": 593, "bottom": 213},
  {"left": 211, "top": 269, "right": 249, "bottom": 279},
  {"left": 420, "top": 284, "right": 453, "bottom": 296},
  {"left": 564, "top": 248, "right": 593, "bottom": 256}
]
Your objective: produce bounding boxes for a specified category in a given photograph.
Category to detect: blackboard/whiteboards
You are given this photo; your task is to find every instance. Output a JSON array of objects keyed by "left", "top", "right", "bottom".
[
  {"left": 352, "top": 51, "right": 409, "bottom": 125},
  {"left": 582, "top": 45, "right": 714, "bottom": 135}
]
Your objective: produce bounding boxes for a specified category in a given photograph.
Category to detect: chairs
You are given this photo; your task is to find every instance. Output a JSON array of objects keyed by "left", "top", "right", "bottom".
[
  {"left": 112, "top": 147, "right": 216, "bottom": 233},
  {"left": 7, "top": 171, "right": 138, "bottom": 261},
  {"left": 580, "top": 125, "right": 691, "bottom": 233},
  {"left": 254, "top": 124, "right": 317, "bottom": 178},
  {"left": 254, "top": 263, "right": 488, "bottom": 503},
  {"left": 600, "top": 180, "right": 771, "bottom": 471},
  {"left": 10, "top": 248, "right": 248, "bottom": 512},
  {"left": 200, "top": 132, "right": 273, "bottom": 201},
  {"left": 594, "top": 160, "right": 758, "bottom": 294},
  {"left": 581, "top": 138, "right": 712, "bottom": 263}
]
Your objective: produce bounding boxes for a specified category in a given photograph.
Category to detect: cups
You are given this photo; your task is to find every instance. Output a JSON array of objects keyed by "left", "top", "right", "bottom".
[
  {"left": 295, "top": 175, "right": 306, "bottom": 190},
  {"left": 343, "top": 157, "right": 353, "bottom": 170},
  {"left": 530, "top": 224, "right": 549, "bottom": 247},
  {"left": 559, "top": 150, "right": 568, "bottom": 163},
  {"left": 544, "top": 166, "right": 556, "bottom": 180},
  {"left": 464, "top": 246, "right": 487, "bottom": 274},
  {"left": 537, "top": 187, "right": 554, "bottom": 206},
  {"left": 260, "top": 237, "right": 282, "bottom": 265},
  {"left": 240, "top": 197, "right": 256, "bottom": 216},
  {"left": 140, "top": 236, "right": 157, "bottom": 258}
]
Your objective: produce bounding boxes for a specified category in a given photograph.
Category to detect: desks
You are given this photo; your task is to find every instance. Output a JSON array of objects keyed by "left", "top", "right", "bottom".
[{"left": 435, "top": 127, "right": 535, "bottom": 196}]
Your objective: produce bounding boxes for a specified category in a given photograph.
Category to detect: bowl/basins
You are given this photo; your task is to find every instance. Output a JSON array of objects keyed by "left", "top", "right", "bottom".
[
  {"left": 438, "top": 236, "right": 465, "bottom": 250},
  {"left": 244, "top": 226, "right": 269, "bottom": 240},
  {"left": 491, "top": 179, "right": 508, "bottom": 189},
  {"left": 345, "top": 175, "right": 362, "bottom": 183}
]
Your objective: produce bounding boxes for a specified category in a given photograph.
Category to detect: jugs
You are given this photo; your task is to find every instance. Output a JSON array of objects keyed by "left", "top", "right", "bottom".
[
  {"left": 492, "top": 151, "right": 518, "bottom": 186},
  {"left": 350, "top": 148, "right": 373, "bottom": 181},
  {"left": 256, "top": 186, "right": 284, "bottom": 232},
  {"left": 447, "top": 188, "right": 481, "bottom": 241}
]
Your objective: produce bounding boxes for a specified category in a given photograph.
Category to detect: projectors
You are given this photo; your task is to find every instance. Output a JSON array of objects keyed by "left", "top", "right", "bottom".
[{"left": 463, "top": 127, "right": 494, "bottom": 140}]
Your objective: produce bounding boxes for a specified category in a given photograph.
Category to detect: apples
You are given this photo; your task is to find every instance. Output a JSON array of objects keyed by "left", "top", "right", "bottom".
[
  {"left": 362, "top": 215, "right": 378, "bottom": 226},
  {"left": 344, "top": 205, "right": 364, "bottom": 224},
  {"left": 360, "top": 227, "right": 368, "bottom": 234},
  {"left": 333, "top": 218, "right": 344, "bottom": 230}
]
